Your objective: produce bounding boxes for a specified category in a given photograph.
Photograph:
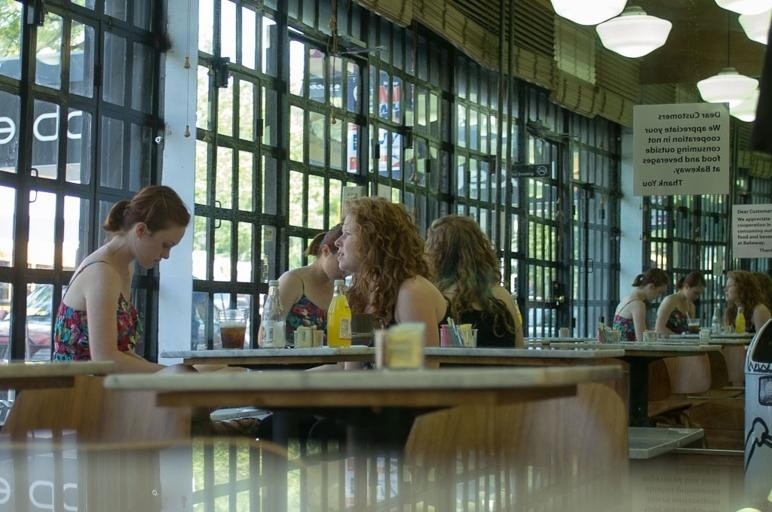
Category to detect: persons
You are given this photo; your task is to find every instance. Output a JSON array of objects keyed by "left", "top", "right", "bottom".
[
  {"left": 724, "top": 270, "right": 772, "bottom": 335},
  {"left": 251, "top": 196, "right": 452, "bottom": 453},
  {"left": 654, "top": 272, "right": 706, "bottom": 429},
  {"left": 257, "top": 222, "right": 378, "bottom": 448},
  {"left": 752, "top": 271, "right": 772, "bottom": 310},
  {"left": 51, "top": 185, "right": 259, "bottom": 439},
  {"left": 612, "top": 268, "right": 670, "bottom": 428},
  {"left": 421, "top": 215, "right": 525, "bottom": 348}
]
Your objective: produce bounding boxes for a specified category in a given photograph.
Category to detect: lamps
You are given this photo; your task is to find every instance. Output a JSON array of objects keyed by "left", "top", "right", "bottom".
[
  {"left": 596, "top": 0, "right": 673, "bottom": 59},
  {"left": 738, "top": 11, "right": 772, "bottom": 47},
  {"left": 715, "top": 0, "right": 772, "bottom": 15},
  {"left": 728, "top": 89, "right": 760, "bottom": 122},
  {"left": 697, "top": 12, "right": 760, "bottom": 108},
  {"left": 553, "top": 0, "right": 628, "bottom": 26}
]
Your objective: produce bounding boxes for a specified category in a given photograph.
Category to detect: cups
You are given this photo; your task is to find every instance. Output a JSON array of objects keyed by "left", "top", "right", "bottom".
[
  {"left": 218, "top": 308, "right": 249, "bottom": 350},
  {"left": 686, "top": 318, "right": 700, "bottom": 334}
]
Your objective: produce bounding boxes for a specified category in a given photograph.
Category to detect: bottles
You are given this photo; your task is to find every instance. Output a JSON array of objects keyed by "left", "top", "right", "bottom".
[
  {"left": 711, "top": 307, "right": 720, "bottom": 335},
  {"left": 512, "top": 294, "right": 523, "bottom": 325},
  {"left": 326, "top": 279, "right": 352, "bottom": 348},
  {"left": 735, "top": 306, "right": 746, "bottom": 334},
  {"left": 260, "top": 279, "right": 286, "bottom": 349}
]
